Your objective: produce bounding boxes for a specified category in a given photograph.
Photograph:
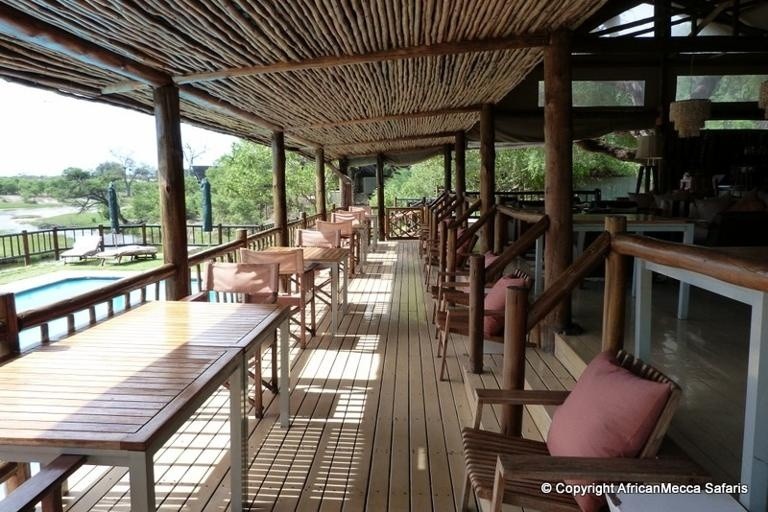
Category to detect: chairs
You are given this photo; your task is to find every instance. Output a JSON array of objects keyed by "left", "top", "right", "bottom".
[
  {"left": 0, "top": 204, "right": 378, "bottom": 512},
  {"left": 417, "top": 190, "right": 534, "bottom": 383},
  {"left": 458, "top": 349, "right": 685, "bottom": 512}
]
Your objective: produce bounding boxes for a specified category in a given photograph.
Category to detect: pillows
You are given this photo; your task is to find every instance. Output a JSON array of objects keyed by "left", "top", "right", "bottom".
[{"left": 545, "top": 351, "right": 671, "bottom": 511}]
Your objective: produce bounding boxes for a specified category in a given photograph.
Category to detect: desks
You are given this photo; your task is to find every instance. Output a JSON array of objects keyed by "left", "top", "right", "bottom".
[{"left": 534, "top": 212, "right": 697, "bottom": 321}]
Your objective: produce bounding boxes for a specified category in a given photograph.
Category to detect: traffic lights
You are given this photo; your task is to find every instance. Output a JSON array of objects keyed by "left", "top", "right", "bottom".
[{"left": 545, "top": 351, "right": 671, "bottom": 511}]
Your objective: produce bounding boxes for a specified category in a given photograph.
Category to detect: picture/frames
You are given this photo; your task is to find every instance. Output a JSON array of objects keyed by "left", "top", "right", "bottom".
[{"left": 534, "top": 212, "right": 697, "bottom": 321}]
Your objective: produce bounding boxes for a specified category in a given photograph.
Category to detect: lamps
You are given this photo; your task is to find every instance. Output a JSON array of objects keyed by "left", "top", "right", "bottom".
[
  {"left": 417, "top": 190, "right": 534, "bottom": 383},
  {"left": 458, "top": 349, "right": 685, "bottom": 512}
]
